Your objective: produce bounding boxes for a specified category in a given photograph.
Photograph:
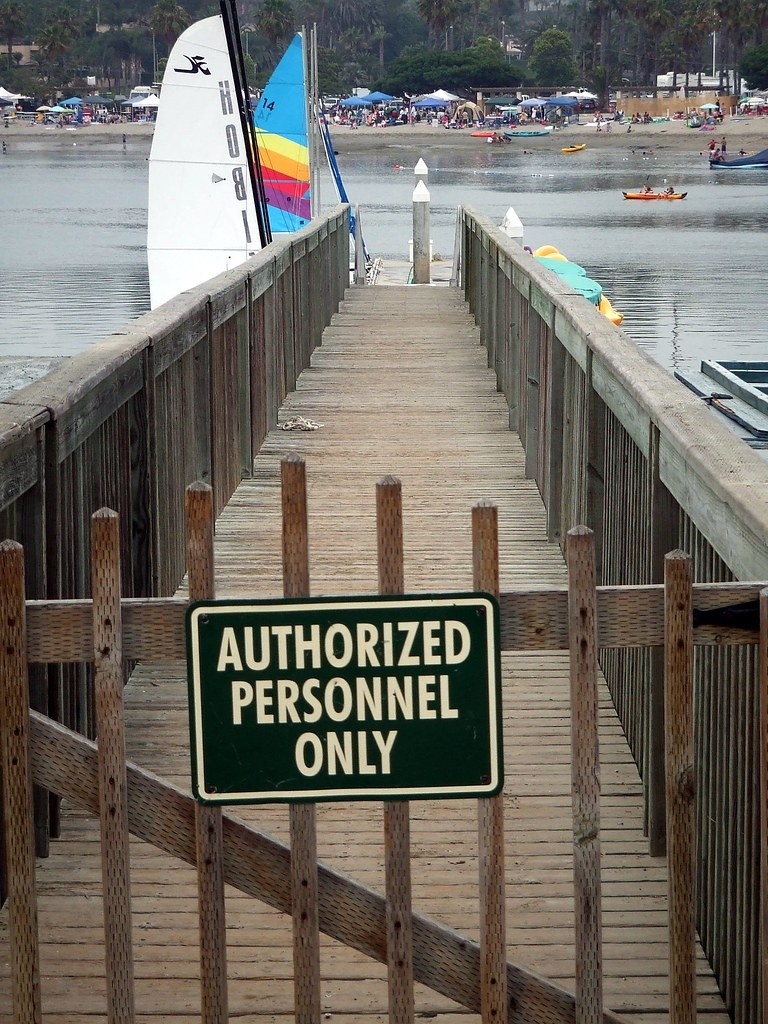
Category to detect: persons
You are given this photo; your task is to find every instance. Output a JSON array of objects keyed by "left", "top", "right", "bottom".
[
  {"left": 3, "top": 141, "right": 6, "bottom": 150},
  {"left": 16, "top": 104, "right": 153, "bottom": 128},
  {"left": 330, "top": 98, "right": 768, "bottom": 195},
  {"left": 122, "top": 134, "right": 126, "bottom": 141}
]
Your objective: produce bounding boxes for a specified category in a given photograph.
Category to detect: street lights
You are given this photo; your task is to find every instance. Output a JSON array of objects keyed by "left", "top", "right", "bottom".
[
  {"left": 592, "top": 42, "right": 601, "bottom": 73},
  {"left": 445, "top": 25, "right": 454, "bottom": 50}
]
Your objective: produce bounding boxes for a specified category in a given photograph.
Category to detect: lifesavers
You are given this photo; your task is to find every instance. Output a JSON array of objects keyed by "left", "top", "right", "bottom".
[{"left": 637, "top": 118, "right": 642, "bottom": 124}]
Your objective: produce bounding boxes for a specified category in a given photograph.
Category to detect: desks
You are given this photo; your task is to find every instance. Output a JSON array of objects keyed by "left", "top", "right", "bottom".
[{"left": 486, "top": 116, "right": 504, "bottom": 128}]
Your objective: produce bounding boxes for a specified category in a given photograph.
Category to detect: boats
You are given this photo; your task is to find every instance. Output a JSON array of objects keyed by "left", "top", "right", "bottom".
[
  {"left": 622, "top": 191, "right": 688, "bottom": 199},
  {"left": 708, "top": 148, "right": 768, "bottom": 168},
  {"left": 471, "top": 132, "right": 493, "bottom": 137},
  {"left": 504, "top": 131, "right": 549, "bottom": 137},
  {"left": 561, "top": 144, "right": 586, "bottom": 152}
]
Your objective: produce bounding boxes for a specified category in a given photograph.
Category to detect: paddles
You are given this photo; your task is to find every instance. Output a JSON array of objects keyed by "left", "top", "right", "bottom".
[
  {"left": 640, "top": 174, "right": 651, "bottom": 193},
  {"left": 657, "top": 189, "right": 669, "bottom": 197}
]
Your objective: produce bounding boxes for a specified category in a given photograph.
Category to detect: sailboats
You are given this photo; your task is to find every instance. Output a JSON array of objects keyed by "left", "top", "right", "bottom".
[
  {"left": 146, "top": 0, "right": 273, "bottom": 312},
  {"left": 247, "top": 22, "right": 374, "bottom": 285}
]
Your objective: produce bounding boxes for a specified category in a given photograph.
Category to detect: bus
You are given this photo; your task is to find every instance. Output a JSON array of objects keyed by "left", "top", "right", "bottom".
[{"left": 129, "top": 86, "right": 159, "bottom": 109}]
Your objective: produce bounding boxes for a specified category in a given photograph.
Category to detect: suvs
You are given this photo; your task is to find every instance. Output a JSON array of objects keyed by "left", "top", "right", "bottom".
[
  {"left": 324, "top": 98, "right": 340, "bottom": 113},
  {"left": 577, "top": 98, "right": 595, "bottom": 114}
]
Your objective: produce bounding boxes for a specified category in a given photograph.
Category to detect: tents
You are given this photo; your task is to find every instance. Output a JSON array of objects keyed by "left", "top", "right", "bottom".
[
  {"left": 485, "top": 96, "right": 521, "bottom": 122},
  {"left": 452, "top": 101, "right": 485, "bottom": 126}
]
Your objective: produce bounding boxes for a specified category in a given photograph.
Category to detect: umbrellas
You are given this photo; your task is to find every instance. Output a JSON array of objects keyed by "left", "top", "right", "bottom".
[
  {"left": 563, "top": 91, "right": 598, "bottom": 113},
  {"left": 423, "top": 89, "right": 460, "bottom": 109},
  {"left": 700, "top": 103, "right": 719, "bottom": 115},
  {"left": 545, "top": 97, "right": 579, "bottom": 124},
  {"left": 410, "top": 98, "right": 450, "bottom": 121},
  {"left": 0, "top": 86, "right": 34, "bottom": 123},
  {"left": 36, "top": 93, "right": 160, "bottom": 123},
  {"left": 518, "top": 98, "right": 547, "bottom": 123},
  {"left": 242, "top": 89, "right": 255, "bottom": 100},
  {"left": 339, "top": 91, "right": 396, "bottom": 121},
  {"left": 739, "top": 96, "right": 764, "bottom": 109}
]
